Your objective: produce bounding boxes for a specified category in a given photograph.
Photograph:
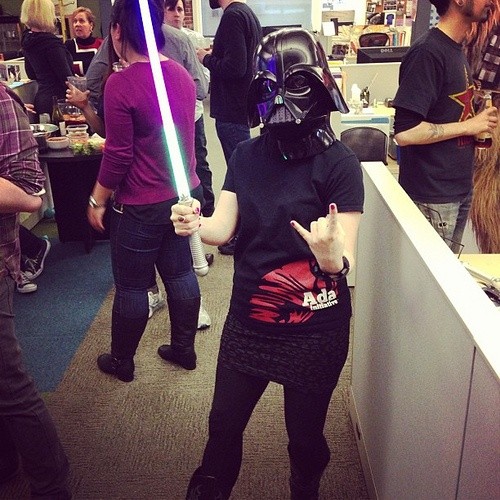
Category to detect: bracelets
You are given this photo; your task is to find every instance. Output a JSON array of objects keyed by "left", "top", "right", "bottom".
[{"left": 308, "top": 257, "right": 351, "bottom": 282}]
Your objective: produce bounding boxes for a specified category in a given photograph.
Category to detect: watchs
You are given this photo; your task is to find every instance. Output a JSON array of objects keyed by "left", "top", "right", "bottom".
[{"left": 87, "top": 194, "right": 107, "bottom": 209}]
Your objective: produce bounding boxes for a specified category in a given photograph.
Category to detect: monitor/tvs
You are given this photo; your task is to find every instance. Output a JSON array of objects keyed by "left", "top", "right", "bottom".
[{"left": 356, "top": 46, "right": 411, "bottom": 63}]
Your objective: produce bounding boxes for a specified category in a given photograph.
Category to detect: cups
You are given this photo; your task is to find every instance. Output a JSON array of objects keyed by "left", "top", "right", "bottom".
[
  {"left": 56, "top": 98, "right": 81, "bottom": 120},
  {"left": 67, "top": 76, "right": 88, "bottom": 101},
  {"left": 67, "top": 133, "right": 89, "bottom": 154},
  {"left": 66, "top": 124, "right": 89, "bottom": 135},
  {"left": 112, "top": 61, "right": 130, "bottom": 73}
]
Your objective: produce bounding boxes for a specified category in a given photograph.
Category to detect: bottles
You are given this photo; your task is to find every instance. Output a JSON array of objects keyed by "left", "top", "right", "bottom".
[
  {"left": 349, "top": 85, "right": 370, "bottom": 114},
  {"left": 474, "top": 91, "right": 493, "bottom": 148},
  {"left": 49, "top": 95, "right": 67, "bottom": 137}
]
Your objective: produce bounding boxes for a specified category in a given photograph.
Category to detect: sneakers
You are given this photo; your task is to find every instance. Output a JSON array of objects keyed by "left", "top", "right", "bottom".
[
  {"left": 218, "top": 234, "right": 238, "bottom": 255},
  {"left": 24, "top": 239, "right": 51, "bottom": 280},
  {"left": 15, "top": 269, "right": 37, "bottom": 293}
]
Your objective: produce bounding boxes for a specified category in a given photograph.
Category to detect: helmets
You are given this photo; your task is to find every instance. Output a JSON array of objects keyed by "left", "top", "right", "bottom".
[{"left": 246, "top": 26, "right": 351, "bottom": 128}]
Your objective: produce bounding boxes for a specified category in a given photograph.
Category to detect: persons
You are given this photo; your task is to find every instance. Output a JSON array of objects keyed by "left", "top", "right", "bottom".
[
  {"left": 83, "top": 0, "right": 215, "bottom": 274},
  {"left": 165, "top": 26, "right": 367, "bottom": 500},
  {"left": 0, "top": 56, "right": 76, "bottom": 500},
  {"left": 197, "top": 0, "right": 264, "bottom": 166},
  {"left": 391, "top": 0, "right": 500, "bottom": 266},
  {"left": 86, "top": 0, "right": 208, "bottom": 382},
  {"left": 65, "top": 16, "right": 212, "bottom": 329},
  {"left": 162, "top": 0, "right": 217, "bottom": 218},
  {"left": 17, "top": 0, "right": 80, "bottom": 129},
  {"left": 62, "top": 6, "right": 108, "bottom": 83}
]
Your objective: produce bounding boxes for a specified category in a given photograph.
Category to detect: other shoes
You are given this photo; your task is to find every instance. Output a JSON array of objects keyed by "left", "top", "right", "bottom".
[
  {"left": 97, "top": 353, "right": 135, "bottom": 382},
  {"left": 148, "top": 288, "right": 165, "bottom": 318},
  {"left": 206, "top": 253, "right": 214, "bottom": 266},
  {"left": 158, "top": 344, "right": 196, "bottom": 370},
  {"left": 197, "top": 304, "right": 211, "bottom": 329}
]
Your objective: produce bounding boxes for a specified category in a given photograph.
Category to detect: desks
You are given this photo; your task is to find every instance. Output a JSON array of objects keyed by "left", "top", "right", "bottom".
[
  {"left": 38, "top": 132, "right": 112, "bottom": 244},
  {"left": 454, "top": 253, "right": 500, "bottom": 315},
  {"left": 341, "top": 99, "right": 389, "bottom": 163}
]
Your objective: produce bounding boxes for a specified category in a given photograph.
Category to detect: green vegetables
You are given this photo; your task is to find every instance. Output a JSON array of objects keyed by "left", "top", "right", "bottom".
[{"left": 74, "top": 143, "right": 93, "bottom": 155}]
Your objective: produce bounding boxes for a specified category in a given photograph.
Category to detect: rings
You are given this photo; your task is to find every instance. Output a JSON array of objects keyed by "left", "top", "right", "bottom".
[{"left": 177, "top": 216, "right": 185, "bottom": 222}]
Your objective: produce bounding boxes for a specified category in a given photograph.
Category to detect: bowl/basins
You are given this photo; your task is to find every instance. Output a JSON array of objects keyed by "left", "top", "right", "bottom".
[
  {"left": 4, "top": 30, "right": 16, "bottom": 39},
  {"left": 46, "top": 137, "right": 69, "bottom": 149},
  {"left": 89, "top": 138, "right": 106, "bottom": 154},
  {"left": 27, "top": 123, "right": 60, "bottom": 153}
]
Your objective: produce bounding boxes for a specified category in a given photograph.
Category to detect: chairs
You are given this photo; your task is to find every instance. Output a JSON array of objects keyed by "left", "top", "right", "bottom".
[
  {"left": 358, "top": 33, "right": 389, "bottom": 47},
  {"left": 340, "top": 128, "right": 388, "bottom": 168}
]
